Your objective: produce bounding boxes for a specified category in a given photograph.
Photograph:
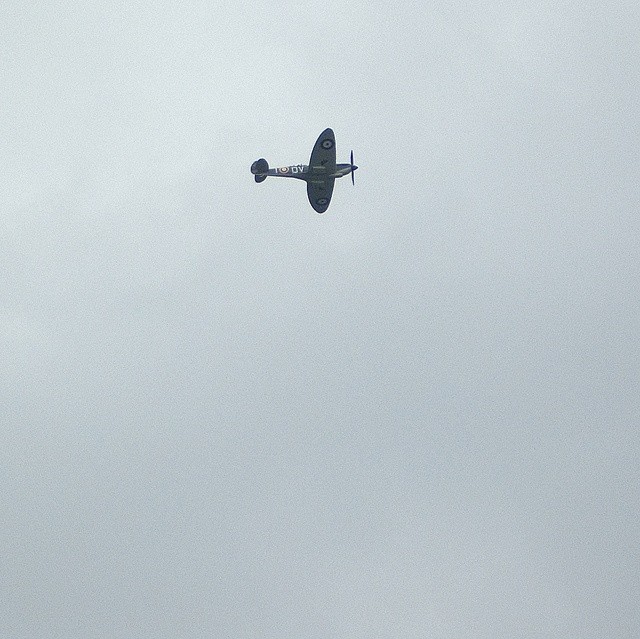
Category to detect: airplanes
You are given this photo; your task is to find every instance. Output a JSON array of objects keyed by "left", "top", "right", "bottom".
[{"left": 250, "top": 127, "right": 359, "bottom": 213}]
